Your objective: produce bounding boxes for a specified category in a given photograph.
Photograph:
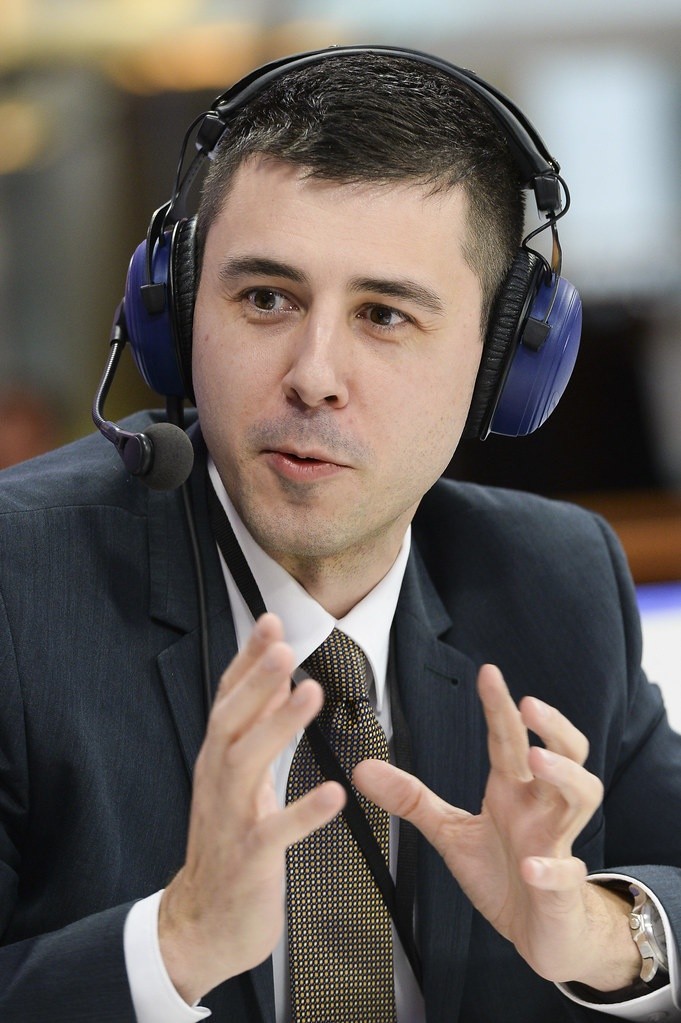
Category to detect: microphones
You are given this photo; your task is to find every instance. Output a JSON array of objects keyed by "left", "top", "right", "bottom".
[{"left": 90, "top": 294, "right": 194, "bottom": 494}]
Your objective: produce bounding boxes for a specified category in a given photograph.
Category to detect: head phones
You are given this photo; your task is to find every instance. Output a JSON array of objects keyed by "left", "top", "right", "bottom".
[{"left": 127, "top": 46, "right": 584, "bottom": 457}]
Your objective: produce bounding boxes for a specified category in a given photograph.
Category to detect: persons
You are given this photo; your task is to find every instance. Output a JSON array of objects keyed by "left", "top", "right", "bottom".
[{"left": 0, "top": 45, "right": 681, "bottom": 1022}]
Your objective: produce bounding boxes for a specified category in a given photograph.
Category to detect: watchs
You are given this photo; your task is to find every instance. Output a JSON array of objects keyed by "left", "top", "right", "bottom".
[{"left": 563, "top": 876, "right": 671, "bottom": 1008}]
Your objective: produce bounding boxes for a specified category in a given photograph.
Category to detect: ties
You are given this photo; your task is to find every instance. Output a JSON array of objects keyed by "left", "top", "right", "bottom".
[{"left": 284, "top": 629, "right": 391, "bottom": 1022}]
